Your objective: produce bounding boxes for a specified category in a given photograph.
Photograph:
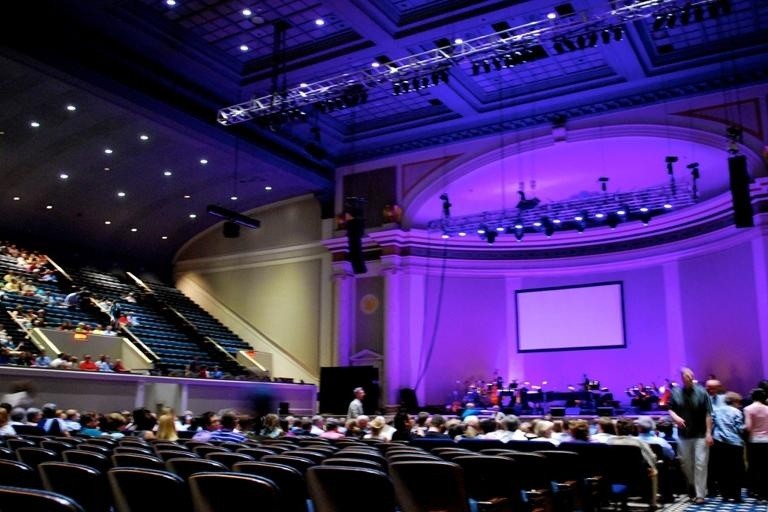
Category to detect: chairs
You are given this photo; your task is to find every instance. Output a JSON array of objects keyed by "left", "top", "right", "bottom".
[
  {"left": 0, "top": 424, "right": 681, "bottom": 512},
  {"left": 0, "top": 251, "right": 254, "bottom": 377}
]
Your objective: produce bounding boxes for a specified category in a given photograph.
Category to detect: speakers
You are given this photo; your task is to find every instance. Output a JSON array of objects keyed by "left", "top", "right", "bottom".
[
  {"left": 223, "top": 222, "right": 240, "bottom": 238},
  {"left": 597, "top": 407, "right": 613, "bottom": 416},
  {"left": 347, "top": 219, "right": 368, "bottom": 273},
  {"left": 400, "top": 388, "right": 419, "bottom": 415},
  {"left": 550, "top": 407, "right": 565, "bottom": 416},
  {"left": 728, "top": 156, "right": 754, "bottom": 228}
]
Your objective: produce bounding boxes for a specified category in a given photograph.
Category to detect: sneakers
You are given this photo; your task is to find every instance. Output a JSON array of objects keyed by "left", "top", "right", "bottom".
[{"left": 687, "top": 495, "right": 705, "bottom": 504}]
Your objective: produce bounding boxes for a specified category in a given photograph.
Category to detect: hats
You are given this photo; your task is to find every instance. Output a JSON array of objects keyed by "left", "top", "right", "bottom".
[{"left": 367, "top": 414, "right": 386, "bottom": 429}]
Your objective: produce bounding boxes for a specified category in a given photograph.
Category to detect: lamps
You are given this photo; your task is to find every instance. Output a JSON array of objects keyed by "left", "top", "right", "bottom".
[{"left": 207, "top": 0, "right": 754, "bottom": 242}]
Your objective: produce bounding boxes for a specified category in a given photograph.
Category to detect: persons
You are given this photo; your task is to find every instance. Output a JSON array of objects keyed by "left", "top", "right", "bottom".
[
  {"left": 347, "top": 387, "right": 365, "bottom": 419},
  {"left": 2, "top": 402, "right": 616, "bottom": 444},
  {"left": 607, "top": 366, "right": 713, "bottom": 503},
  {"left": 461, "top": 376, "right": 528, "bottom": 408},
  {"left": 577, "top": 374, "right": 590, "bottom": 390},
  {"left": 705, "top": 379, "right": 768, "bottom": 503},
  {"left": 0, "top": 239, "right": 147, "bottom": 375}
]
